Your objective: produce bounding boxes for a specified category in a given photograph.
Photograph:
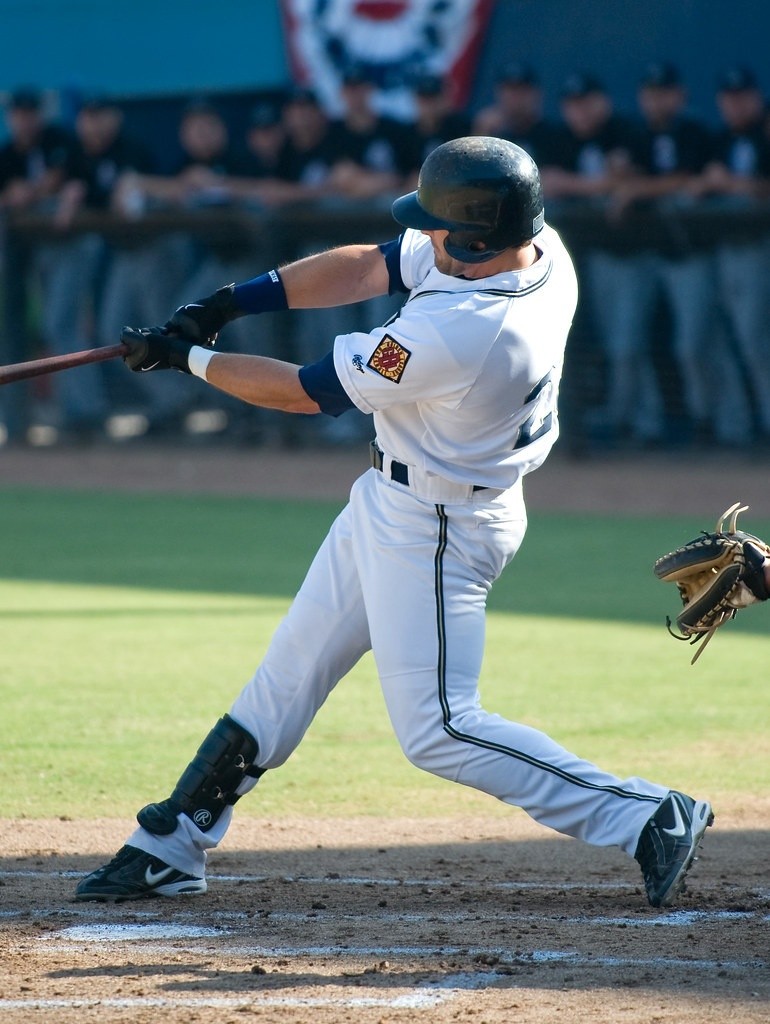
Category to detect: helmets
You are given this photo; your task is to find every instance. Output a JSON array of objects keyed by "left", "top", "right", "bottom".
[{"left": 391, "top": 135, "right": 545, "bottom": 264}]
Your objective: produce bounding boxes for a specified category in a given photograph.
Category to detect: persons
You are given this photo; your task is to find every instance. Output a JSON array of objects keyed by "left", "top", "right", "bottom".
[
  {"left": 70, "top": 136, "right": 716, "bottom": 907},
  {"left": 0, "top": 72, "right": 418, "bottom": 446},
  {"left": 393, "top": 63, "right": 770, "bottom": 453}
]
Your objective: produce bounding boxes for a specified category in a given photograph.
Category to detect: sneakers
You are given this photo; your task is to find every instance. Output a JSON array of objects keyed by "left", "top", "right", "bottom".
[
  {"left": 634, "top": 790, "right": 713, "bottom": 908},
  {"left": 75, "top": 844, "right": 208, "bottom": 900}
]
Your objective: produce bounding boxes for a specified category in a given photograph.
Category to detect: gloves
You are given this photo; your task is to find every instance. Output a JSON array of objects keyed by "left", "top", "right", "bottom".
[
  {"left": 120, "top": 284, "right": 195, "bottom": 375},
  {"left": 162, "top": 295, "right": 227, "bottom": 347}
]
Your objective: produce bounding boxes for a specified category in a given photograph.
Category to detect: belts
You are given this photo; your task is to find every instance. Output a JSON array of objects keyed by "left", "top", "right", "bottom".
[{"left": 369, "top": 441, "right": 489, "bottom": 492}]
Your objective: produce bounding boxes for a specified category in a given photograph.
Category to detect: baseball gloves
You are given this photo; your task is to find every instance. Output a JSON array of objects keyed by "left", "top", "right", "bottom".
[{"left": 652, "top": 530, "right": 770, "bottom": 636}]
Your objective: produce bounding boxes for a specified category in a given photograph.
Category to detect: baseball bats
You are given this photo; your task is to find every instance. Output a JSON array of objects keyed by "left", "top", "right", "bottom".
[{"left": 0, "top": 332, "right": 217, "bottom": 385}]
